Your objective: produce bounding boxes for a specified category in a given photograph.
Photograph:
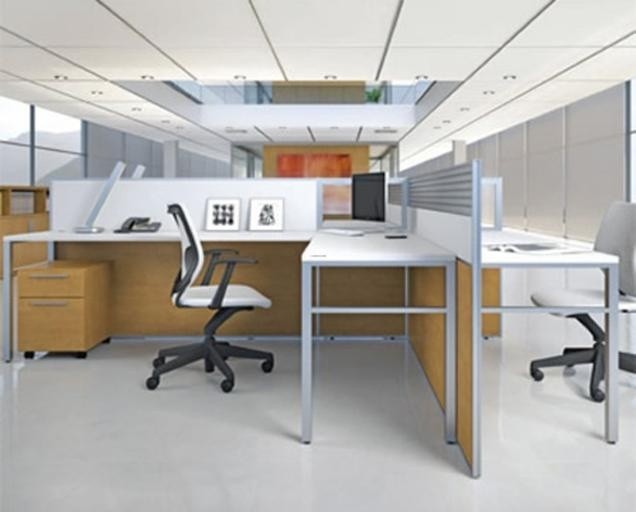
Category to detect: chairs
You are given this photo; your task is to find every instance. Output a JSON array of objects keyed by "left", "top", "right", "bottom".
[
  {"left": 529, "top": 201, "right": 636, "bottom": 403},
  {"left": 146, "top": 201, "right": 274, "bottom": 394}
]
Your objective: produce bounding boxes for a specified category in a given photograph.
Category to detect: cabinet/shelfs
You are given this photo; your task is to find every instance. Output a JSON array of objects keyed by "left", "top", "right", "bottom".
[
  {"left": 0, "top": 184, "right": 49, "bottom": 280},
  {"left": 17, "top": 260, "right": 111, "bottom": 359}
]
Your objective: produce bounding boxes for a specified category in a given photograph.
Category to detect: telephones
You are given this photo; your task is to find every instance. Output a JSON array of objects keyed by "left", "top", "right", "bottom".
[{"left": 122, "top": 217, "right": 161, "bottom": 232}]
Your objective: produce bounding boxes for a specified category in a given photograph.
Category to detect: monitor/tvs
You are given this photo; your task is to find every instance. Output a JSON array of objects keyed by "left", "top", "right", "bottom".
[{"left": 352, "top": 171, "right": 387, "bottom": 232}]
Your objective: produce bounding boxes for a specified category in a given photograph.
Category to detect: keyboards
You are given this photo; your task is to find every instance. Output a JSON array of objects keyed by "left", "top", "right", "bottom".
[{"left": 321, "top": 228, "right": 364, "bottom": 236}]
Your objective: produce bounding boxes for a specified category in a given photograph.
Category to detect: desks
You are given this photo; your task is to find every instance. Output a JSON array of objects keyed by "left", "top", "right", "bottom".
[
  {"left": 480, "top": 227, "right": 620, "bottom": 444},
  {"left": 3, "top": 221, "right": 457, "bottom": 445}
]
